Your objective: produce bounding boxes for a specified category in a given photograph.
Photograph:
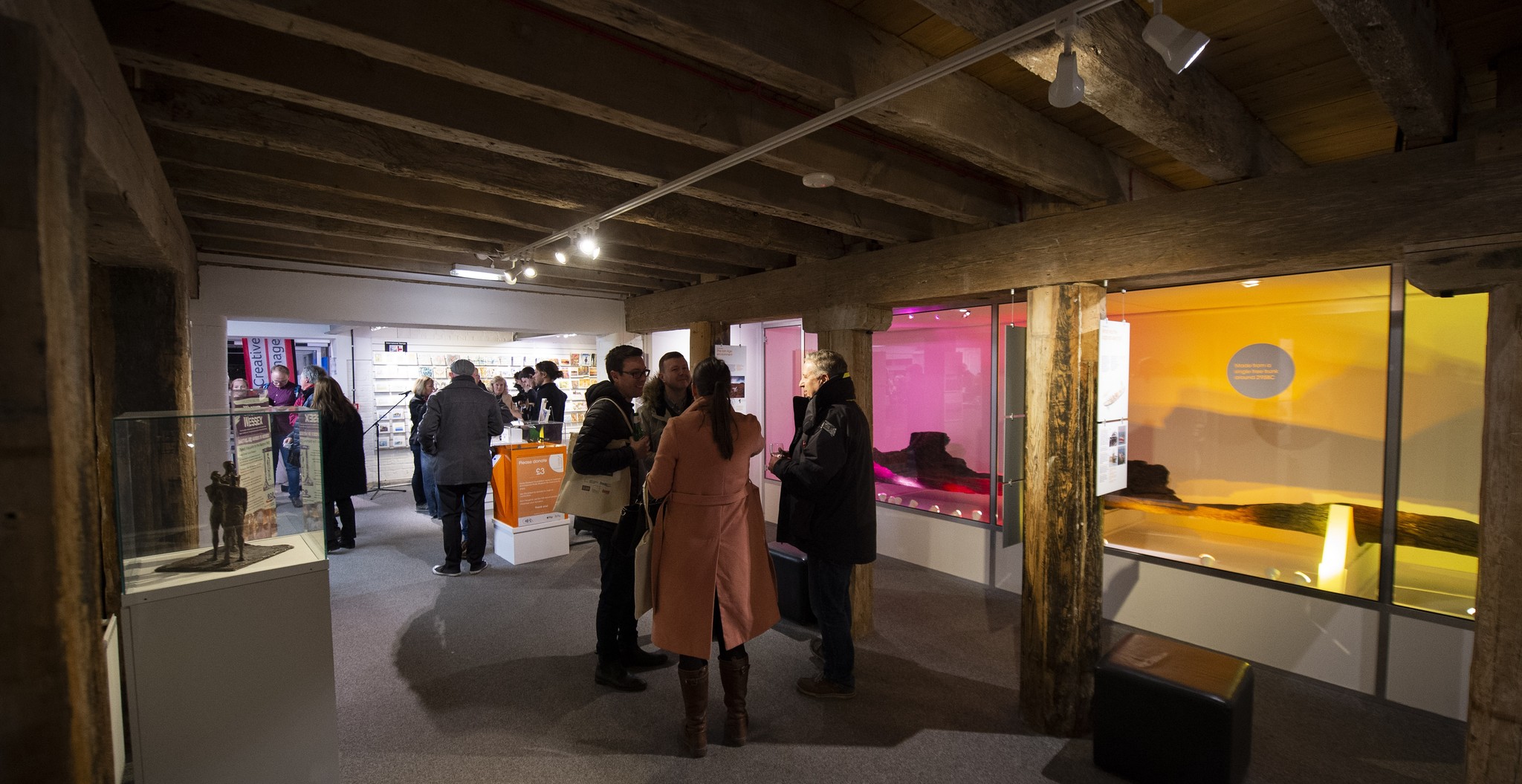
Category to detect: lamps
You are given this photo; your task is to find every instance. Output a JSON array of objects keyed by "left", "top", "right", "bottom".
[
  {"left": 1048, "top": 14, "right": 1086, "bottom": 107},
  {"left": 450, "top": 263, "right": 506, "bottom": 282},
  {"left": 1143, "top": 1, "right": 1211, "bottom": 75},
  {"left": 1264, "top": 567, "right": 1280, "bottom": 580},
  {"left": 1199, "top": 553, "right": 1216, "bottom": 567},
  {"left": 504, "top": 249, "right": 538, "bottom": 284},
  {"left": 972, "top": 510, "right": 982, "bottom": 521},
  {"left": 928, "top": 504, "right": 940, "bottom": 513},
  {"left": 909, "top": 499, "right": 918, "bottom": 508},
  {"left": 555, "top": 231, "right": 578, "bottom": 263},
  {"left": 888, "top": 496, "right": 895, "bottom": 504},
  {"left": 894, "top": 497, "right": 902, "bottom": 505},
  {"left": 577, "top": 221, "right": 600, "bottom": 260},
  {"left": 949, "top": 509, "right": 961, "bottom": 517},
  {"left": 879, "top": 494, "right": 886, "bottom": 502},
  {"left": 1290, "top": 570, "right": 1311, "bottom": 586}
]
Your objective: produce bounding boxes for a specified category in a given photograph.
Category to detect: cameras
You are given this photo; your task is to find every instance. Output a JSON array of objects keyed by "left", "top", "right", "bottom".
[{"left": 512, "top": 383, "right": 528, "bottom": 403}]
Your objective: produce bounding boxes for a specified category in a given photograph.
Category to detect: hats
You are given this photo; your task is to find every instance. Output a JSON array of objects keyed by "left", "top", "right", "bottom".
[{"left": 451, "top": 359, "right": 474, "bottom": 376}]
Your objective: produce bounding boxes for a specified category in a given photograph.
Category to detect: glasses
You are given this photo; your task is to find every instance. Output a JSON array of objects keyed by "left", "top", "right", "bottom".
[
  {"left": 272, "top": 379, "right": 288, "bottom": 384},
  {"left": 615, "top": 369, "right": 651, "bottom": 379}
]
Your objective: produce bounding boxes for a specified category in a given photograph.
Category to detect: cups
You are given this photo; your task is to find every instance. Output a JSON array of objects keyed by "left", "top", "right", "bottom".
[{"left": 770, "top": 443, "right": 784, "bottom": 459}]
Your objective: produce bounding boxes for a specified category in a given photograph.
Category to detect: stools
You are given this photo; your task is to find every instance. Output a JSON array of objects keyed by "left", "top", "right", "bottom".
[
  {"left": 767, "top": 541, "right": 814, "bottom": 626},
  {"left": 1091, "top": 630, "right": 1255, "bottom": 784}
]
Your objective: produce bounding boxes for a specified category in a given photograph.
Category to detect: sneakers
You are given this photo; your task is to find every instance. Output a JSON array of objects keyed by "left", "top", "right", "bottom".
[
  {"left": 469, "top": 560, "right": 488, "bottom": 574},
  {"left": 809, "top": 637, "right": 824, "bottom": 663},
  {"left": 796, "top": 670, "right": 857, "bottom": 699},
  {"left": 432, "top": 565, "right": 461, "bottom": 576},
  {"left": 416, "top": 502, "right": 429, "bottom": 512}
]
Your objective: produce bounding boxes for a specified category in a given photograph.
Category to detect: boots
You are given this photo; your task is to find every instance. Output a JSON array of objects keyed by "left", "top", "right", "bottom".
[
  {"left": 718, "top": 652, "right": 749, "bottom": 747},
  {"left": 677, "top": 659, "right": 709, "bottom": 758}
]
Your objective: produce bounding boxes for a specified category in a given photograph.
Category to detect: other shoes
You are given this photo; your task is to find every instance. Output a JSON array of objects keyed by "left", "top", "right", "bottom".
[
  {"left": 327, "top": 538, "right": 340, "bottom": 552},
  {"left": 338, "top": 538, "right": 356, "bottom": 549},
  {"left": 594, "top": 666, "right": 648, "bottom": 692},
  {"left": 461, "top": 540, "right": 468, "bottom": 558},
  {"left": 618, "top": 648, "right": 668, "bottom": 668}
]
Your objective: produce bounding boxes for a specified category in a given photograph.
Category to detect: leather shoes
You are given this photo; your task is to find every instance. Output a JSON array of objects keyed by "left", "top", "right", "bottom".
[{"left": 290, "top": 497, "right": 303, "bottom": 507}]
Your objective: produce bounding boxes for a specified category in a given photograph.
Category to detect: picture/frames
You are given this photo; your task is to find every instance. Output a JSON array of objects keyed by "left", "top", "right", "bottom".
[{"left": 372, "top": 352, "right": 596, "bottom": 449}]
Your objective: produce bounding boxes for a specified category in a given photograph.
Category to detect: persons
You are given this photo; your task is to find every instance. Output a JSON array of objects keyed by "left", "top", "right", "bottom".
[
  {"left": 232, "top": 378, "right": 248, "bottom": 397},
  {"left": 259, "top": 365, "right": 367, "bottom": 551},
  {"left": 768, "top": 350, "right": 876, "bottom": 698},
  {"left": 574, "top": 345, "right": 780, "bottom": 755},
  {"left": 408, "top": 359, "right": 568, "bottom": 577},
  {"left": 206, "top": 461, "right": 247, "bottom": 565}
]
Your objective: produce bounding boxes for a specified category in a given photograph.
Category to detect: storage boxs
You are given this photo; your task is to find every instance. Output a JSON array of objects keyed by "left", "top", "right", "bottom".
[{"left": 501, "top": 428, "right": 523, "bottom": 443}]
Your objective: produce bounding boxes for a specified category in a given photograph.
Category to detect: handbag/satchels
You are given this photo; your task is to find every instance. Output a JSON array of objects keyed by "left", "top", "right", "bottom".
[
  {"left": 634, "top": 473, "right": 655, "bottom": 621},
  {"left": 553, "top": 398, "right": 634, "bottom": 525}
]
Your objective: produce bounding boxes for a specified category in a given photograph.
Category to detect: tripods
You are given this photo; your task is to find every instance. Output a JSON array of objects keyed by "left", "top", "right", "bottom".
[{"left": 363, "top": 394, "right": 408, "bottom": 500}]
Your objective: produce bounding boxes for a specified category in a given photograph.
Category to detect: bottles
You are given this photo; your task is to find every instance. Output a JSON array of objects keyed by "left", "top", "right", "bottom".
[{"left": 632, "top": 413, "right": 645, "bottom": 441}]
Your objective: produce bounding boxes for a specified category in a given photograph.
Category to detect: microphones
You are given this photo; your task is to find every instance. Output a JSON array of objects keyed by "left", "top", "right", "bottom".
[
  {"left": 398, "top": 390, "right": 412, "bottom": 395},
  {"left": 529, "top": 376, "right": 532, "bottom": 388}
]
